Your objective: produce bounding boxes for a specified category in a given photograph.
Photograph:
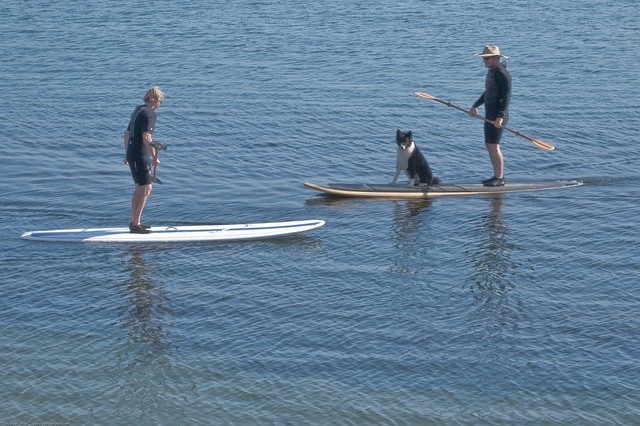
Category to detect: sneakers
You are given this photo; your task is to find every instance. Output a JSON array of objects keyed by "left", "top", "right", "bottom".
[
  {"left": 129, "top": 225, "right": 150, "bottom": 234},
  {"left": 129, "top": 221, "right": 150, "bottom": 229},
  {"left": 481, "top": 177, "right": 495, "bottom": 183},
  {"left": 482, "top": 176, "right": 506, "bottom": 187}
]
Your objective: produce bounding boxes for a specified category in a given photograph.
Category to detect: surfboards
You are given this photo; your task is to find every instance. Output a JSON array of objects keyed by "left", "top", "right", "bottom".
[
  {"left": 20, "top": 219, "right": 325, "bottom": 244},
  {"left": 302, "top": 179, "right": 586, "bottom": 200}
]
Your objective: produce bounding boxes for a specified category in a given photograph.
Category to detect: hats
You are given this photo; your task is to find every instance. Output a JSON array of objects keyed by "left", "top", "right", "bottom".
[{"left": 478, "top": 45, "right": 508, "bottom": 59}]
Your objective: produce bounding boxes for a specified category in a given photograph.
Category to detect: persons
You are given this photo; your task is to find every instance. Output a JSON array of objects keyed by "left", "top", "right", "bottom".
[
  {"left": 469, "top": 46, "right": 512, "bottom": 186},
  {"left": 123, "top": 86, "right": 165, "bottom": 234}
]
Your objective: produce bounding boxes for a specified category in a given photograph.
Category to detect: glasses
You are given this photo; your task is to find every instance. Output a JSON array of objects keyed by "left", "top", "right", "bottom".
[
  {"left": 482, "top": 56, "right": 494, "bottom": 60},
  {"left": 159, "top": 100, "right": 163, "bottom": 104}
]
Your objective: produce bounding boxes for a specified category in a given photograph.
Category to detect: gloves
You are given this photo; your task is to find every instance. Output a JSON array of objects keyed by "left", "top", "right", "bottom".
[{"left": 149, "top": 140, "right": 167, "bottom": 151}]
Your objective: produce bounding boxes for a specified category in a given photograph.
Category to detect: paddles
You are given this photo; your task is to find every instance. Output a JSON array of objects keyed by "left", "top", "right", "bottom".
[
  {"left": 151, "top": 141, "right": 167, "bottom": 186},
  {"left": 414, "top": 89, "right": 556, "bottom": 153}
]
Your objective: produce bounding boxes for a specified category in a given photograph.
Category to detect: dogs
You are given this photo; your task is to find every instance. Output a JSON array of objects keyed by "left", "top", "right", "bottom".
[{"left": 390, "top": 129, "right": 441, "bottom": 190}]
